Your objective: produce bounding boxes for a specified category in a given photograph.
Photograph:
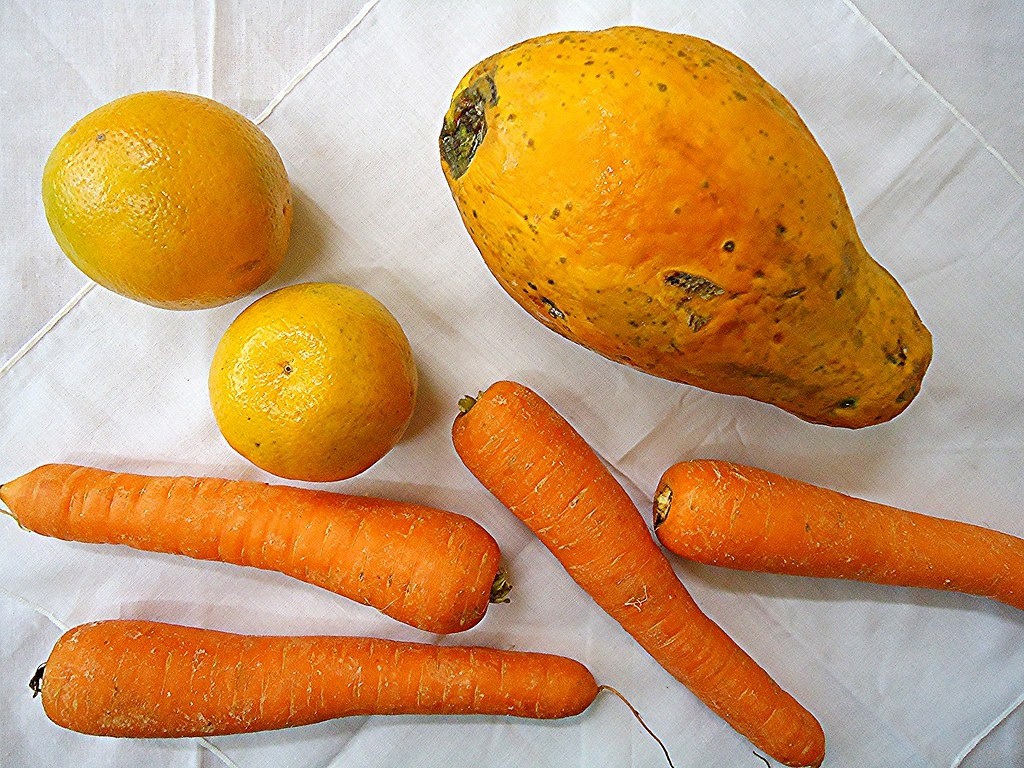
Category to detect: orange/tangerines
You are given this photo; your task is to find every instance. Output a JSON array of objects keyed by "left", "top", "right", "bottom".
[
  {"left": 208, "top": 281, "right": 418, "bottom": 481},
  {"left": 41, "top": 91, "right": 292, "bottom": 313}
]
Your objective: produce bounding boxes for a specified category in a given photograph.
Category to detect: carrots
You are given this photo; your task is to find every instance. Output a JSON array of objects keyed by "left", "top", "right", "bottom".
[
  {"left": 450, "top": 379, "right": 826, "bottom": 768},
  {"left": 653, "top": 457, "right": 1024, "bottom": 613},
  {"left": 0, "top": 464, "right": 513, "bottom": 635},
  {"left": 29, "top": 617, "right": 603, "bottom": 739}
]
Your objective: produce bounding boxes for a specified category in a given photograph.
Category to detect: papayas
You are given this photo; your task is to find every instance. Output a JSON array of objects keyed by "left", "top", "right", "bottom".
[{"left": 440, "top": 26, "right": 933, "bottom": 431}]
